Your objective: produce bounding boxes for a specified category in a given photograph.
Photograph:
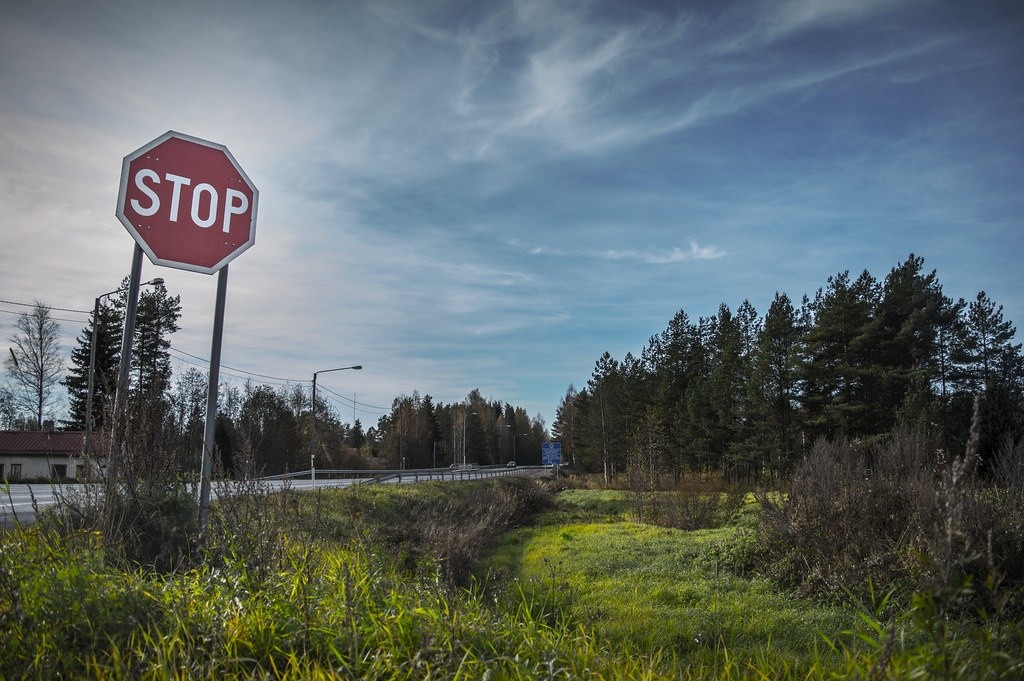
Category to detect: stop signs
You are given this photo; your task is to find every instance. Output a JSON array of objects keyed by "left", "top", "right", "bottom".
[{"left": 115, "top": 129, "right": 260, "bottom": 276}]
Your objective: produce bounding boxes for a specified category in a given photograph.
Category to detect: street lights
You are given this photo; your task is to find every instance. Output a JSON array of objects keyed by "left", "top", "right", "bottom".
[
  {"left": 312, "top": 366, "right": 362, "bottom": 469},
  {"left": 83, "top": 277, "right": 164, "bottom": 484},
  {"left": 396, "top": 397, "right": 528, "bottom": 475}
]
